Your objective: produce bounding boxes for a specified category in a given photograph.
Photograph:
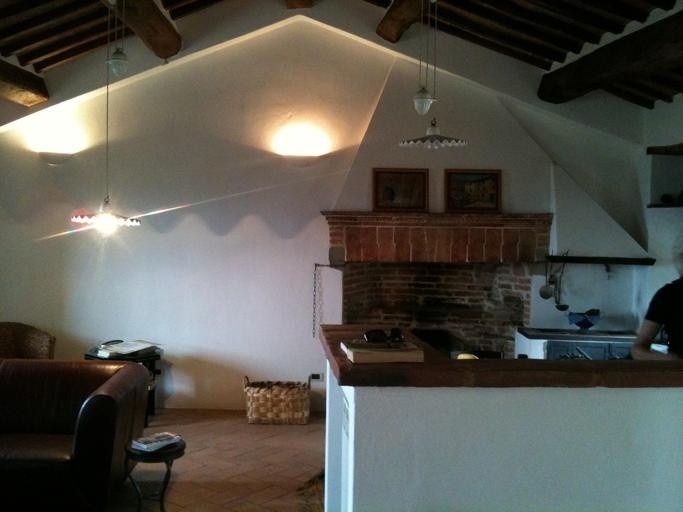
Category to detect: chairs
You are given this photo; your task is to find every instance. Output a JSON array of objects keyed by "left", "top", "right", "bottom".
[{"left": 0, "top": 321, "right": 56, "bottom": 359}]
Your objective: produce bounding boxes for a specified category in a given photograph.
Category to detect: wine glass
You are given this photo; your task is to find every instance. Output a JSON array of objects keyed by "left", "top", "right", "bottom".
[{"left": 568, "top": 311, "right": 600, "bottom": 334}]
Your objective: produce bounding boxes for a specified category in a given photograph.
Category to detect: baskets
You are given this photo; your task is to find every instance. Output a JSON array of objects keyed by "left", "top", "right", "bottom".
[{"left": 241, "top": 374, "right": 312, "bottom": 425}]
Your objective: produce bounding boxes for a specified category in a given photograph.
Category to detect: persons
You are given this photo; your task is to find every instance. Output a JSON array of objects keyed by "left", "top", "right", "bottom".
[{"left": 630, "top": 275, "right": 683, "bottom": 360}]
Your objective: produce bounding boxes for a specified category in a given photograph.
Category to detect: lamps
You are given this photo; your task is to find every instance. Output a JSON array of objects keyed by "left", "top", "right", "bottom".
[
  {"left": 398, "top": 0, "right": 468, "bottom": 150},
  {"left": 412, "top": 0, "right": 434, "bottom": 114},
  {"left": 70, "top": 0, "right": 140, "bottom": 236},
  {"left": 110, "top": 0, "right": 129, "bottom": 76}
]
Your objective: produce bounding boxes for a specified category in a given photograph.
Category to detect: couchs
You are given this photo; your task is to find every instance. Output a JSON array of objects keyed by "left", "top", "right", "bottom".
[{"left": 0, "top": 357, "right": 150, "bottom": 494}]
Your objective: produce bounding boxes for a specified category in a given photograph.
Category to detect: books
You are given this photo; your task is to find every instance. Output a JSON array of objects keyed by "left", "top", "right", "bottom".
[
  {"left": 97, "top": 339, "right": 160, "bottom": 359},
  {"left": 131, "top": 431, "right": 182, "bottom": 452},
  {"left": 340, "top": 341, "right": 424, "bottom": 365}
]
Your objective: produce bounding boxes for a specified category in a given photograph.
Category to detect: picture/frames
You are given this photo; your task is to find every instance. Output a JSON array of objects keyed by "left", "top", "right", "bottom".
[
  {"left": 444, "top": 168, "right": 502, "bottom": 214},
  {"left": 372, "top": 167, "right": 430, "bottom": 213}
]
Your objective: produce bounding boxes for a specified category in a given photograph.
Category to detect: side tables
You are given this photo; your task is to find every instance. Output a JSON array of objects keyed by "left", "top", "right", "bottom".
[{"left": 123, "top": 437, "right": 186, "bottom": 511}]
